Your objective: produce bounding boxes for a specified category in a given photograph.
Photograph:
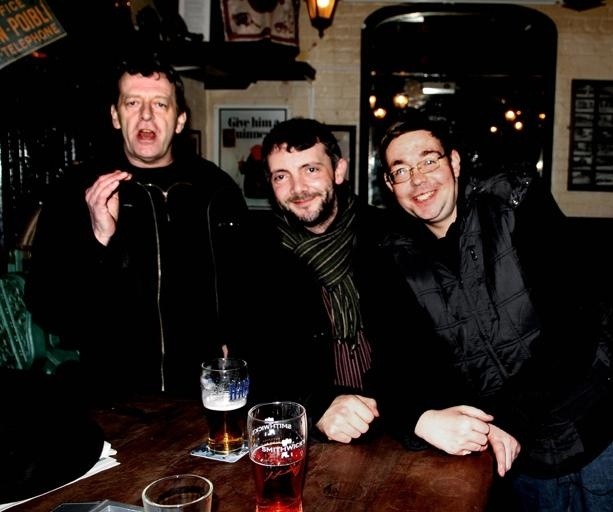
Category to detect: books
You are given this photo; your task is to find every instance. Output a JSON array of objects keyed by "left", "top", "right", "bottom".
[{"left": 1, "top": 416, "right": 123, "bottom": 512}]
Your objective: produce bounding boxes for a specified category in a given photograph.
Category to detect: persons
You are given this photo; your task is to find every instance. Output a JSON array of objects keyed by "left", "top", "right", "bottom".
[
  {"left": 358, "top": 113, "right": 613, "bottom": 512},
  {"left": 22, "top": 56, "right": 248, "bottom": 405},
  {"left": 217, "top": 112, "right": 384, "bottom": 445}
]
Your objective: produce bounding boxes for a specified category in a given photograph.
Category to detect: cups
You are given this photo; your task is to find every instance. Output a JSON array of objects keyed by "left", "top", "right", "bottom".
[
  {"left": 246, "top": 401, "right": 307, "bottom": 512},
  {"left": 199, "top": 357, "right": 249, "bottom": 456},
  {"left": 141, "top": 473, "right": 213, "bottom": 512}
]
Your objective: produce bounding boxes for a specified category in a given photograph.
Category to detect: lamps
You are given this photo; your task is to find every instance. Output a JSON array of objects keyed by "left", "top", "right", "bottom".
[{"left": 305, "top": 0, "right": 339, "bottom": 38}]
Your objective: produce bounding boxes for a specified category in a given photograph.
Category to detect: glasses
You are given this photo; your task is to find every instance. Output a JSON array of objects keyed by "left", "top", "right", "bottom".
[{"left": 387, "top": 154, "right": 445, "bottom": 185}]
